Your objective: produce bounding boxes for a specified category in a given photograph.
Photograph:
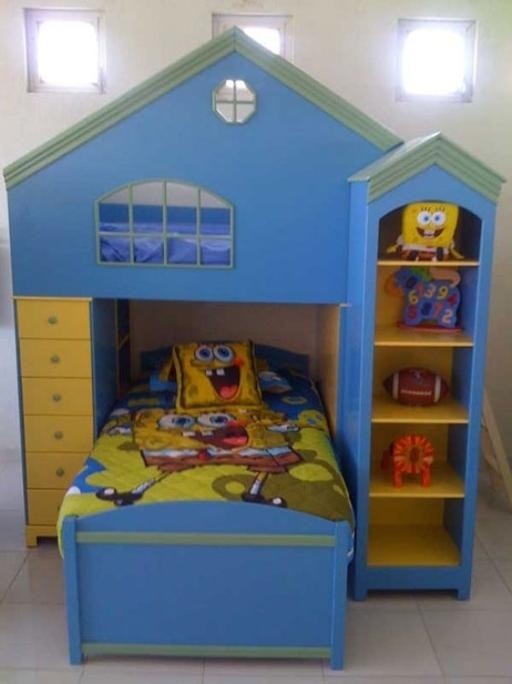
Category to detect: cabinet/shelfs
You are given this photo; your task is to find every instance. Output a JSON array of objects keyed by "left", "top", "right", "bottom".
[
  {"left": 15, "top": 296, "right": 94, "bottom": 526},
  {"left": 367, "top": 199, "right": 481, "bottom": 568}
]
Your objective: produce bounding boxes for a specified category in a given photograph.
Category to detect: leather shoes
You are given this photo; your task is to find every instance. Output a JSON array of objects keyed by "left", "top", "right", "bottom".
[
  {"left": 241, "top": 487, "right": 289, "bottom": 511},
  {"left": 96, "top": 484, "right": 146, "bottom": 509}
]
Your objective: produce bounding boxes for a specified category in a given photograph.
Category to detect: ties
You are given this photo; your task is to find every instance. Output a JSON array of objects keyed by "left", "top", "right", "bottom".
[{"left": 193, "top": 447, "right": 214, "bottom": 464}]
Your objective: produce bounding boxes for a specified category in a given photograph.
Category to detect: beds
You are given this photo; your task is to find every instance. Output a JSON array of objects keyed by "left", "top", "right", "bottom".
[{"left": 58, "top": 338, "right": 356, "bottom": 670}]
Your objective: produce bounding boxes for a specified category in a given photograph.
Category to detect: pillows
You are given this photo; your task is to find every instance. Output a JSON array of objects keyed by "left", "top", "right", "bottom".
[{"left": 172, "top": 338, "right": 265, "bottom": 413}]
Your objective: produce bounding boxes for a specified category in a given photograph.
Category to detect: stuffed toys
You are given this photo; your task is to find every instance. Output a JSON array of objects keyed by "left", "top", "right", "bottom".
[
  {"left": 386, "top": 200, "right": 466, "bottom": 260},
  {"left": 385, "top": 265, "right": 462, "bottom": 332}
]
